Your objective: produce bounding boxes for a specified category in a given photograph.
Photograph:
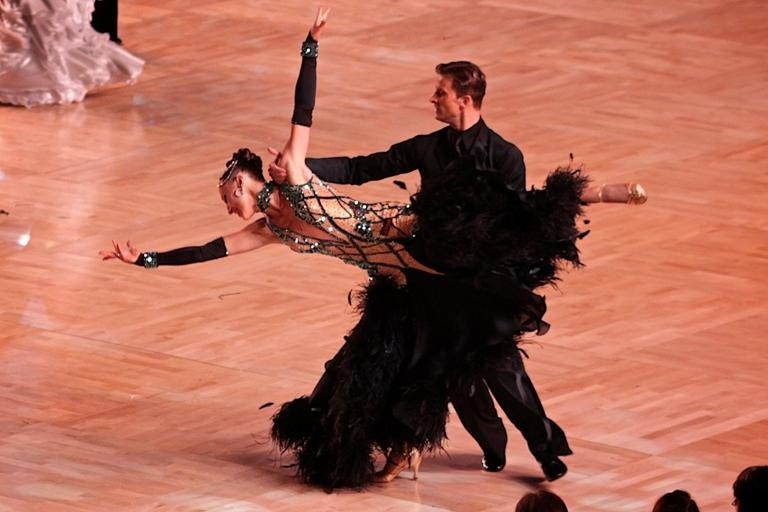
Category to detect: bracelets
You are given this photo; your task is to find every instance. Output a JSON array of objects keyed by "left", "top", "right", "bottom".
[
  {"left": 298, "top": 41, "right": 319, "bottom": 59},
  {"left": 142, "top": 249, "right": 160, "bottom": 271}
]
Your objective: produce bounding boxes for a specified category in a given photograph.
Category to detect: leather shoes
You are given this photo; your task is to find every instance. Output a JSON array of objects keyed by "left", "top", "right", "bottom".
[
  {"left": 481, "top": 455, "right": 506, "bottom": 472},
  {"left": 541, "top": 457, "right": 568, "bottom": 482}
]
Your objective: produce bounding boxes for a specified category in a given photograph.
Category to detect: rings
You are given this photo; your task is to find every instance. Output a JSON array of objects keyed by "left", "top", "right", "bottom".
[{"left": 115, "top": 253, "right": 120, "bottom": 258}]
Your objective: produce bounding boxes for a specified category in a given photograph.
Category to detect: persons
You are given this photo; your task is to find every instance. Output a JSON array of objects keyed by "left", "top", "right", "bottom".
[
  {"left": 512, "top": 491, "right": 568, "bottom": 512},
  {"left": 728, "top": 465, "right": 767, "bottom": 512},
  {"left": 93, "top": 6, "right": 650, "bottom": 495},
  {"left": 263, "top": 59, "right": 571, "bottom": 486},
  {"left": 89, "top": 0, "right": 123, "bottom": 47},
  {"left": 650, "top": 490, "right": 702, "bottom": 512},
  {"left": 0, "top": 0, "right": 147, "bottom": 112}
]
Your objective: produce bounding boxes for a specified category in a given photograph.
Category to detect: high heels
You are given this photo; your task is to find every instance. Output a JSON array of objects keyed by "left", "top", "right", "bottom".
[
  {"left": 366, "top": 447, "right": 421, "bottom": 484},
  {"left": 598, "top": 184, "right": 648, "bottom": 205}
]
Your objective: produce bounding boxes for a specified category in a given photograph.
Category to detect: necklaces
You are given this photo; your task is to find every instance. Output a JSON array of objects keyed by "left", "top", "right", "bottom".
[{"left": 256, "top": 178, "right": 275, "bottom": 214}]
